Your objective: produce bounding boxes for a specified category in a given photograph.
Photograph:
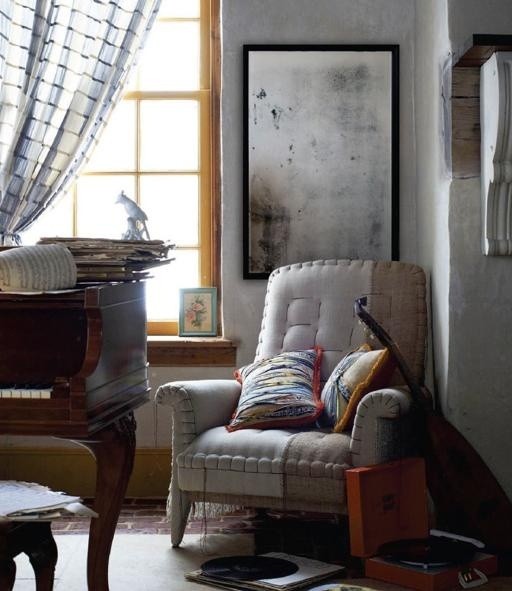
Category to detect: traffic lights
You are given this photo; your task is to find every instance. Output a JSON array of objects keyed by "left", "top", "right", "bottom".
[
  {"left": 225, "top": 346, "right": 325, "bottom": 432},
  {"left": 314, "top": 343, "right": 395, "bottom": 433}
]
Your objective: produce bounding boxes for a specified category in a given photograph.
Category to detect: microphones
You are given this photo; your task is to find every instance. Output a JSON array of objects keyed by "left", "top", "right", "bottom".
[{"left": 0, "top": 521, "right": 58, "bottom": 591}]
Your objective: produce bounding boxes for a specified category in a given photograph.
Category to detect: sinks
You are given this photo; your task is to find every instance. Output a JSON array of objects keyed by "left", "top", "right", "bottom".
[{"left": 153, "top": 259, "right": 429, "bottom": 548}]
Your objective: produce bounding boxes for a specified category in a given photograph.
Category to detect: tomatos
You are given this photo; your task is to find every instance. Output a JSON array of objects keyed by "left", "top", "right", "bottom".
[{"left": 0, "top": 281, "right": 151, "bottom": 591}]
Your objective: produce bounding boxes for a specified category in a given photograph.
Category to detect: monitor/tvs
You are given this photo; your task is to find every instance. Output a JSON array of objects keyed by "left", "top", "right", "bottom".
[
  {"left": 183, "top": 549, "right": 346, "bottom": 591},
  {"left": 0, "top": 236, "right": 176, "bottom": 295}
]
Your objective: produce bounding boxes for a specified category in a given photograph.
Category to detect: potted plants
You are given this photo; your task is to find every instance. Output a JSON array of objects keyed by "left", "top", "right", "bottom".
[
  {"left": 179, "top": 288, "right": 217, "bottom": 336},
  {"left": 243, "top": 44, "right": 400, "bottom": 280}
]
